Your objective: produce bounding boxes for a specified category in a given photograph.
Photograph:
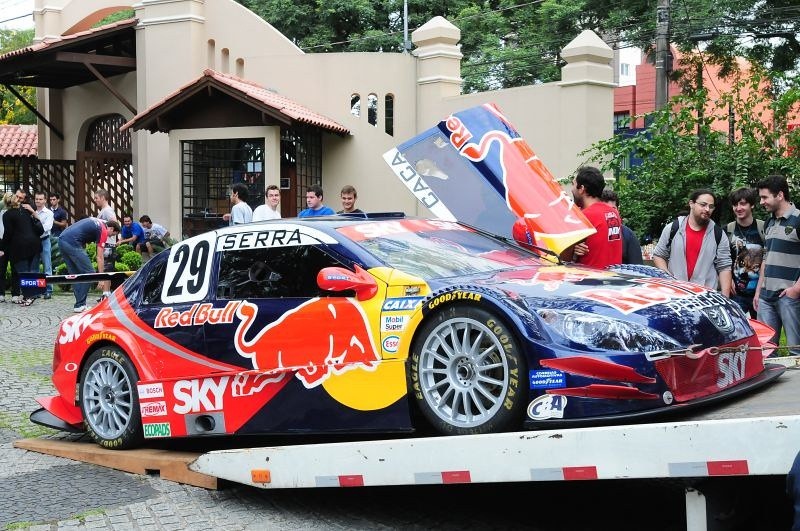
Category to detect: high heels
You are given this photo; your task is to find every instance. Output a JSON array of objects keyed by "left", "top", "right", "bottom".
[
  {"left": 14, "top": 297, "right": 28, "bottom": 304},
  {"left": 21, "top": 295, "right": 41, "bottom": 306}
]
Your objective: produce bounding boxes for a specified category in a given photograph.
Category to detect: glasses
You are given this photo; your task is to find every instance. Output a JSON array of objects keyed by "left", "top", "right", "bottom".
[{"left": 694, "top": 201, "right": 716, "bottom": 210}]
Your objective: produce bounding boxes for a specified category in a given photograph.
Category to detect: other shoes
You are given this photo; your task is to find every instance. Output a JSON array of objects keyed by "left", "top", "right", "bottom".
[
  {"left": 12, "top": 296, "right": 19, "bottom": 302},
  {"left": 44, "top": 293, "right": 52, "bottom": 299},
  {"left": 74, "top": 305, "right": 90, "bottom": 313},
  {"left": 0, "top": 295, "right": 5, "bottom": 302}
]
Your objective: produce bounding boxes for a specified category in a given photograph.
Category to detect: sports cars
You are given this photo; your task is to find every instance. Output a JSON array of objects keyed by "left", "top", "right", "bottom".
[{"left": 18, "top": 103, "right": 787, "bottom": 449}]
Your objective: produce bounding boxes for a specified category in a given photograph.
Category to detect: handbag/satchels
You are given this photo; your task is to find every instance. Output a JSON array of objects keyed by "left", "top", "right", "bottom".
[{"left": 29, "top": 214, "right": 45, "bottom": 236}]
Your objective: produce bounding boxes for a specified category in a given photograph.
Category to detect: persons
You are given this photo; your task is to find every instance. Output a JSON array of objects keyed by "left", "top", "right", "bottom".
[
  {"left": 93, "top": 189, "right": 117, "bottom": 303},
  {"left": 115, "top": 215, "right": 144, "bottom": 248},
  {"left": 753, "top": 175, "right": 800, "bottom": 358},
  {"left": 721, "top": 188, "right": 765, "bottom": 320},
  {"left": 48, "top": 193, "right": 68, "bottom": 237},
  {"left": 223, "top": 183, "right": 253, "bottom": 226},
  {"left": 560, "top": 167, "right": 622, "bottom": 266},
  {"left": 58, "top": 217, "right": 121, "bottom": 313},
  {"left": 135, "top": 215, "right": 170, "bottom": 258},
  {"left": 297, "top": 184, "right": 336, "bottom": 217},
  {"left": 652, "top": 189, "right": 732, "bottom": 300},
  {"left": 336, "top": 185, "right": 365, "bottom": 218},
  {"left": 252, "top": 185, "right": 281, "bottom": 221},
  {"left": 0, "top": 188, "right": 54, "bottom": 305},
  {"left": 600, "top": 190, "right": 643, "bottom": 264}
]
